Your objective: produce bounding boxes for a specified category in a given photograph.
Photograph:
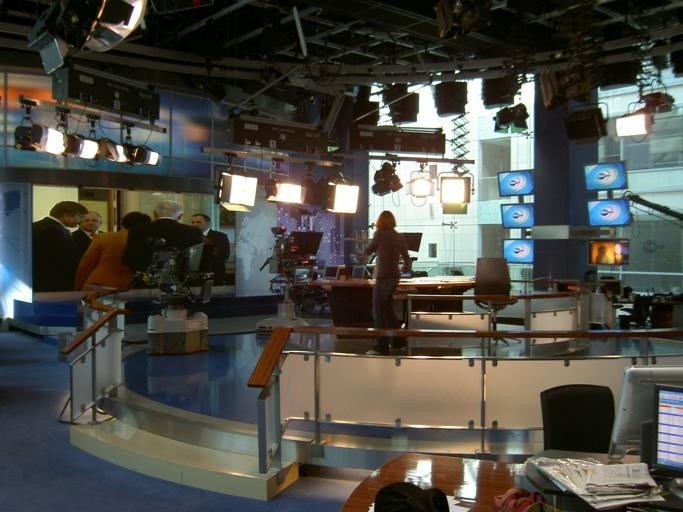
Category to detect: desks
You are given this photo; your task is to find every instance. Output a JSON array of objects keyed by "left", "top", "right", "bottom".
[
  {"left": 305, "top": 277, "right": 477, "bottom": 340},
  {"left": 339, "top": 452, "right": 533, "bottom": 512},
  {"left": 614, "top": 299, "right": 683, "bottom": 328}
]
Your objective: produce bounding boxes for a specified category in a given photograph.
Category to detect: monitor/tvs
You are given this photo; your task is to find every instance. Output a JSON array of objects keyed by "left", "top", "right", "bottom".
[
  {"left": 607, "top": 365, "right": 683, "bottom": 461},
  {"left": 581, "top": 160, "right": 628, "bottom": 192},
  {"left": 585, "top": 197, "right": 632, "bottom": 226},
  {"left": 497, "top": 169, "right": 534, "bottom": 197},
  {"left": 651, "top": 383, "right": 683, "bottom": 499},
  {"left": 500, "top": 203, "right": 534, "bottom": 228},
  {"left": 502, "top": 239, "right": 535, "bottom": 263},
  {"left": 324, "top": 265, "right": 339, "bottom": 280},
  {"left": 588, "top": 238, "right": 630, "bottom": 266}
]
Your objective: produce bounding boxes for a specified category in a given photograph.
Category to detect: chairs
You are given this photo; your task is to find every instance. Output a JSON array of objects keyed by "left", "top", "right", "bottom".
[
  {"left": 541, "top": 384, "right": 615, "bottom": 454},
  {"left": 618, "top": 296, "right": 656, "bottom": 328},
  {"left": 474, "top": 257, "right": 522, "bottom": 347}
]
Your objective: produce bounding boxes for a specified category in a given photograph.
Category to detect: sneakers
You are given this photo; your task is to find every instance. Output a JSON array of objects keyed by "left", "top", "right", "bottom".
[{"left": 365, "top": 344, "right": 405, "bottom": 354}]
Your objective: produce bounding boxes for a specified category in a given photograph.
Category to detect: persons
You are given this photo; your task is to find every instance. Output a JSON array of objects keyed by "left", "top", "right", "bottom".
[
  {"left": 32, "top": 200, "right": 232, "bottom": 291},
  {"left": 365, "top": 210, "right": 413, "bottom": 357}
]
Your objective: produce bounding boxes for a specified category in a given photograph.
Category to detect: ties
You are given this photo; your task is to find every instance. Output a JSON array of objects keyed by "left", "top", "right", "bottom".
[{"left": 89, "top": 233, "right": 97, "bottom": 238}]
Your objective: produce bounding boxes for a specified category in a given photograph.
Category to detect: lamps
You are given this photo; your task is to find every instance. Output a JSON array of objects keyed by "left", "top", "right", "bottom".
[
  {"left": 537, "top": 70, "right": 674, "bottom": 144},
  {"left": 352, "top": 70, "right": 530, "bottom": 134},
  {"left": 15, "top": 94, "right": 161, "bottom": 166},
  {"left": 212, "top": 150, "right": 360, "bottom": 214},
  {"left": 26, "top": 1, "right": 148, "bottom": 74},
  {"left": 372, "top": 153, "right": 470, "bottom": 203}
]
